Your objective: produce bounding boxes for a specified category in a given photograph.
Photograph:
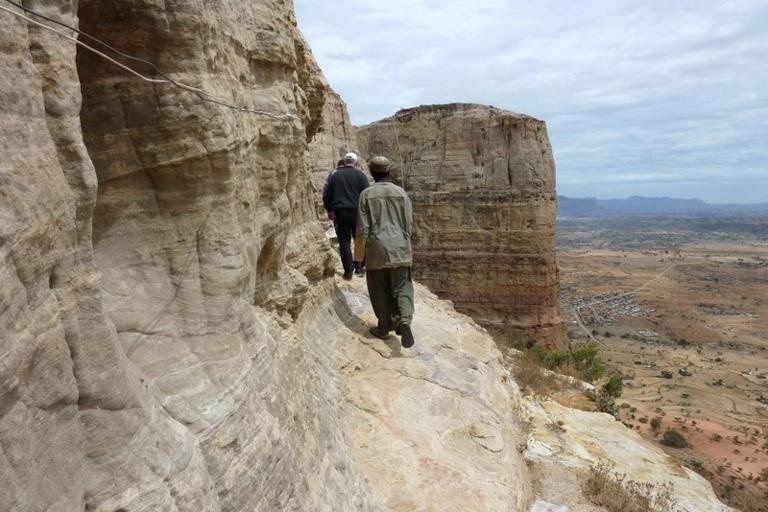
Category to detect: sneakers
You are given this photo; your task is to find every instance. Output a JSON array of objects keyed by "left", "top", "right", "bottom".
[
  {"left": 342, "top": 270, "right": 365, "bottom": 280},
  {"left": 370, "top": 326, "right": 389, "bottom": 339},
  {"left": 399, "top": 321, "right": 415, "bottom": 348}
]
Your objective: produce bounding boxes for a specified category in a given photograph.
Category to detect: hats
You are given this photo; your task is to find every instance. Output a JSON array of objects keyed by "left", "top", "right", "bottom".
[
  {"left": 344, "top": 152, "right": 357, "bottom": 164},
  {"left": 367, "top": 156, "right": 391, "bottom": 173}
]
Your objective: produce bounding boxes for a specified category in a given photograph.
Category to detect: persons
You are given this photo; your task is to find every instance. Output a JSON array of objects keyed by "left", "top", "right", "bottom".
[
  {"left": 352, "top": 155, "right": 415, "bottom": 348},
  {"left": 322, "top": 159, "right": 344, "bottom": 197},
  {"left": 323, "top": 152, "right": 369, "bottom": 280}
]
